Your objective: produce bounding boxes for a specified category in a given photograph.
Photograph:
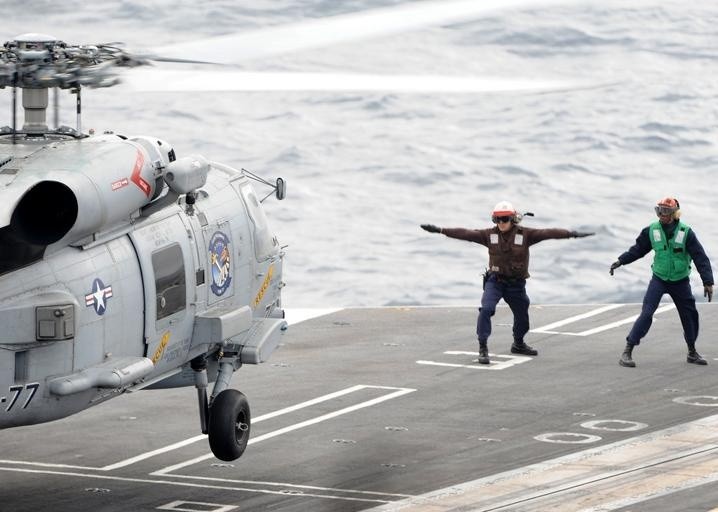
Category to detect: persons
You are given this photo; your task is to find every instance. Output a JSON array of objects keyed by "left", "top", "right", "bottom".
[
  {"left": 418, "top": 203, "right": 596, "bottom": 365},
  {"left": 608, "top": 196, "right": 716, "bottom": 369}
]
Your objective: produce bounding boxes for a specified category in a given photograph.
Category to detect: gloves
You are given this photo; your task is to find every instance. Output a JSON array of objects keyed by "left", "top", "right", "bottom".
[
  {"left": 609, "top": 261, "right": 622, "bottom": 275},
  {"left": 569, "top": 231, "right": 595, "bottom": 238},
  {"left": 421, "top": 225, "right": 441, "bottom": 233},
  {"left": 704, "top": 284, "right": 713, "bottom": 302}
]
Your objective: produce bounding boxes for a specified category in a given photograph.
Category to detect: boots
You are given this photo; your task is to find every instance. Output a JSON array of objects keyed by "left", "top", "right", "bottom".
[
  {"left": 619, "top": 343, "right": 636, "bottom": 367},
  {"left": 477, "top": 343, "right": 489, "bottom": 364},
  {"left": 511, "top": 337, "right": 537, "bottom": 355},
  {"left": 687, "top": 342, "right": 707, "bottom": 366}
]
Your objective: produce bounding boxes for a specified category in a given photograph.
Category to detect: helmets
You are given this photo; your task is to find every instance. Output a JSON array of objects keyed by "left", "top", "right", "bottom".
[
  {"left": 491, "top": 201, "right": 516, "bottom": 217},
  {"left": 658, "top": 197, "right": 680, "bottom": 207}
]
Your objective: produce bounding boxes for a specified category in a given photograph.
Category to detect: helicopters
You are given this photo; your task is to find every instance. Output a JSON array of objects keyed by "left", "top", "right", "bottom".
[{"left": 0, "top": 0, "right": 566, "bottom": 464}]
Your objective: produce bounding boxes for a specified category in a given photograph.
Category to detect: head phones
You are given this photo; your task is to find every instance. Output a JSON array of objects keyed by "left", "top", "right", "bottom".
[
  {"left": 673, "top": 198, "right": 681, "bottom": 220},
  {"left": 492, "top": 214, "right": 522, "bottom": 223}
]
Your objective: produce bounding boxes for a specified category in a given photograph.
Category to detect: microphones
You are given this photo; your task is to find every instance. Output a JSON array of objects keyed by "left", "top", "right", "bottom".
[{"left": 524, "top": 213, "right": 534, "bottom": 216}]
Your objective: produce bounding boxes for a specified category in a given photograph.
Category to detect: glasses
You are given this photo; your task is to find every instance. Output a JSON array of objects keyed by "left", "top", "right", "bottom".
[
  {"left": 655, "top": 206, "right": 679, "bottom": 215},
  {"left": 492, "top": 216, "right": 513, "bottom": 223}
]
